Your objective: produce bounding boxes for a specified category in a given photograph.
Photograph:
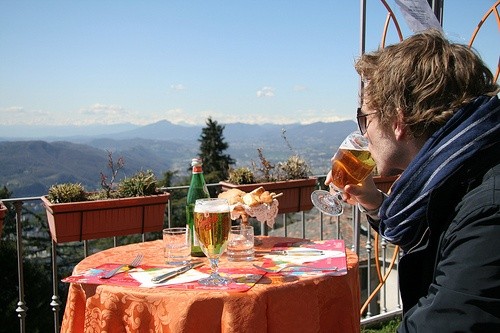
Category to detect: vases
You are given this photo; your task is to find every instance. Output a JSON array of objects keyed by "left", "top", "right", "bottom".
[
  {"left": 371, "top": 172, "right": 403, "bottom": 193},
  {"left": 0, "top": 202, "right": 8, "bottom": 239}
]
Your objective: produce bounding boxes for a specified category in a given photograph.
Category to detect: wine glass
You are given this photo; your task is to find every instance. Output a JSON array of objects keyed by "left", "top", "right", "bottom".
[
  {"left": 193, "top": 196, "right": 233, "bottom": 286},
  {"left": 311, "top": 131, "right": 376, "bottom": 216}
]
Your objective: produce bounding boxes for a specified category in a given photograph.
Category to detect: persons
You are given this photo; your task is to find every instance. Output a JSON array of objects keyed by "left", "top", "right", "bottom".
[{"left": 324, "top": 31, "right": 500, "bottom": 333}]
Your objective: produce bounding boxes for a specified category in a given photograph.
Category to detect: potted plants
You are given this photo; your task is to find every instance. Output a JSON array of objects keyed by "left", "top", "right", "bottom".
[
  {"left": 40, "top": 148, "right": 172, "bottom": 246},
  {"left": 219, "top": 128, "right": 319, "bottom": 216}
]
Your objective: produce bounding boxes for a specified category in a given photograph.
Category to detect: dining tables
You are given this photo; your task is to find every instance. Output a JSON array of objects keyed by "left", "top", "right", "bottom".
[{"left": 58, "top": 231, "right": 361, "bottom": 333}]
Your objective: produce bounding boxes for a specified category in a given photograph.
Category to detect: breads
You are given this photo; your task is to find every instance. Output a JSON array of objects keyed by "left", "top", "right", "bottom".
[{"left": 218, "top": 186, "right": 273, "bottom": 208}]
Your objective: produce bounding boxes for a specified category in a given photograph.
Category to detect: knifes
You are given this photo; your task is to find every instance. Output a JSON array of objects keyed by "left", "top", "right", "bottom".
[
  {"left": 151, "top": 261, "right": 205, "bottom": 284},
  {"left": 254, "top": 250, "right": 322, "bottom": 255}
]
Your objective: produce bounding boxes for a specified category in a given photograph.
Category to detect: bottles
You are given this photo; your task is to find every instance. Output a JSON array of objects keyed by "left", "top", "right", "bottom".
[{"left": 186, "top": 157, "right": 210, "bottom": 257}]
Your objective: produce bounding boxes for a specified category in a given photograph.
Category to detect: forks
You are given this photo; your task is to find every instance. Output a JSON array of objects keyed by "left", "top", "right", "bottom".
[
  {"left": 97, "top": 252, "right": 144, "bottom": 280},
  {"left": 253, "top": 263, "right": 337, "bottom": 274}
]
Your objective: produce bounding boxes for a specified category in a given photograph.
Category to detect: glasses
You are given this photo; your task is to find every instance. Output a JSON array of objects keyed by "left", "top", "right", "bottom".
[{"left": 356, "top": 107, "right": 381, "bottom": 135}]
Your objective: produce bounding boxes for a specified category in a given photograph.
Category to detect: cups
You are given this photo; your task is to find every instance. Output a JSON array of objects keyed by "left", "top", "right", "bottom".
[
  {"left": 162, "top": 228, "right": 192, "bottom": 264},
  {"left": 227, "top": 225, "right": 255, "bottom": 262}
]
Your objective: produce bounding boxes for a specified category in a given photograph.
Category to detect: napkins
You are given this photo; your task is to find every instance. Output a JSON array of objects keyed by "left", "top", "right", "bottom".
[
  {"left": 261, "top": 245, "right": 345, "bottom": 264},
  {"left": 127, "top": 266, "right": 209, "bottom": 288}
]
